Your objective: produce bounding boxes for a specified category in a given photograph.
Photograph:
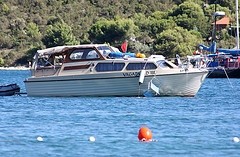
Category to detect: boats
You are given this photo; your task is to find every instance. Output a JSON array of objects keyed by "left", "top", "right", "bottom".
[{"left": 24, "top": 45, "right": 208, "bottom": 98}]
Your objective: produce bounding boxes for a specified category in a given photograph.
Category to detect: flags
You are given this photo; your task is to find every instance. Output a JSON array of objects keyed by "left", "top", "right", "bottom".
[{"left": 121, "top": 41, "right": 128, "bottom": 52}]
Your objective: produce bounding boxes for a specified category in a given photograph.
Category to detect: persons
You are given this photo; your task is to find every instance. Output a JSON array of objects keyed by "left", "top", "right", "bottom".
[{"left": 193, "top": 48, "right": 223, "bottom": 66}]
